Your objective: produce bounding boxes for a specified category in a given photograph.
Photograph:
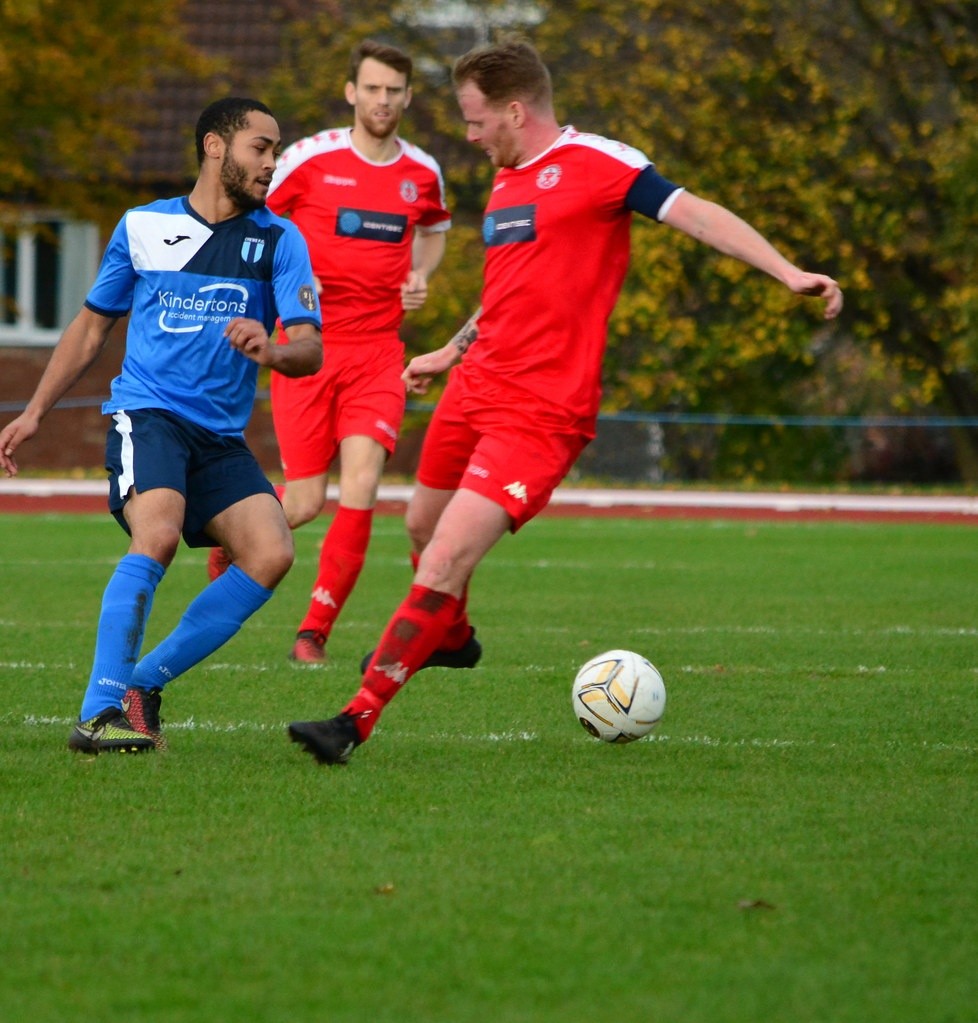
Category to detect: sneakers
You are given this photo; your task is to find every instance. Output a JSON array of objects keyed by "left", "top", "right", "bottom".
[
  {"left": 67, "top": 706, "right": 155, "bottom": 756},
  {"left": 288, "top": 707, "right": 362, "bottom": 767},
  {"left": 208, "top": 545, "right": 233, "bottom": 583},
  {"left": 360, "top": 622, "right": 482, "bottom": 676},
  {"left": 119, "top": 686, "right": 168, "bottom": 754},
  {"left": 288, "top": 638, "right": 330, "bottom": 668}
]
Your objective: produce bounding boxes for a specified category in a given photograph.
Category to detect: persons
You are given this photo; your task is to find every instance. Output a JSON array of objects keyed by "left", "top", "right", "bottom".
[
  {"left": 280, "top": 41, "right": 844, "bottom": 766},
  {"left": 0, "top": 95, "right": 327, "bottom": 752},
  {"left": 204, "top": 46, "right": 454, "bottom": 673}
]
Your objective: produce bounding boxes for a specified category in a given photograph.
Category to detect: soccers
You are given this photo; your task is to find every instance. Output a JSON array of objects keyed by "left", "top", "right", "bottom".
[{"left": 571, "top": 649, "right": 668, "bottom": 743}]
